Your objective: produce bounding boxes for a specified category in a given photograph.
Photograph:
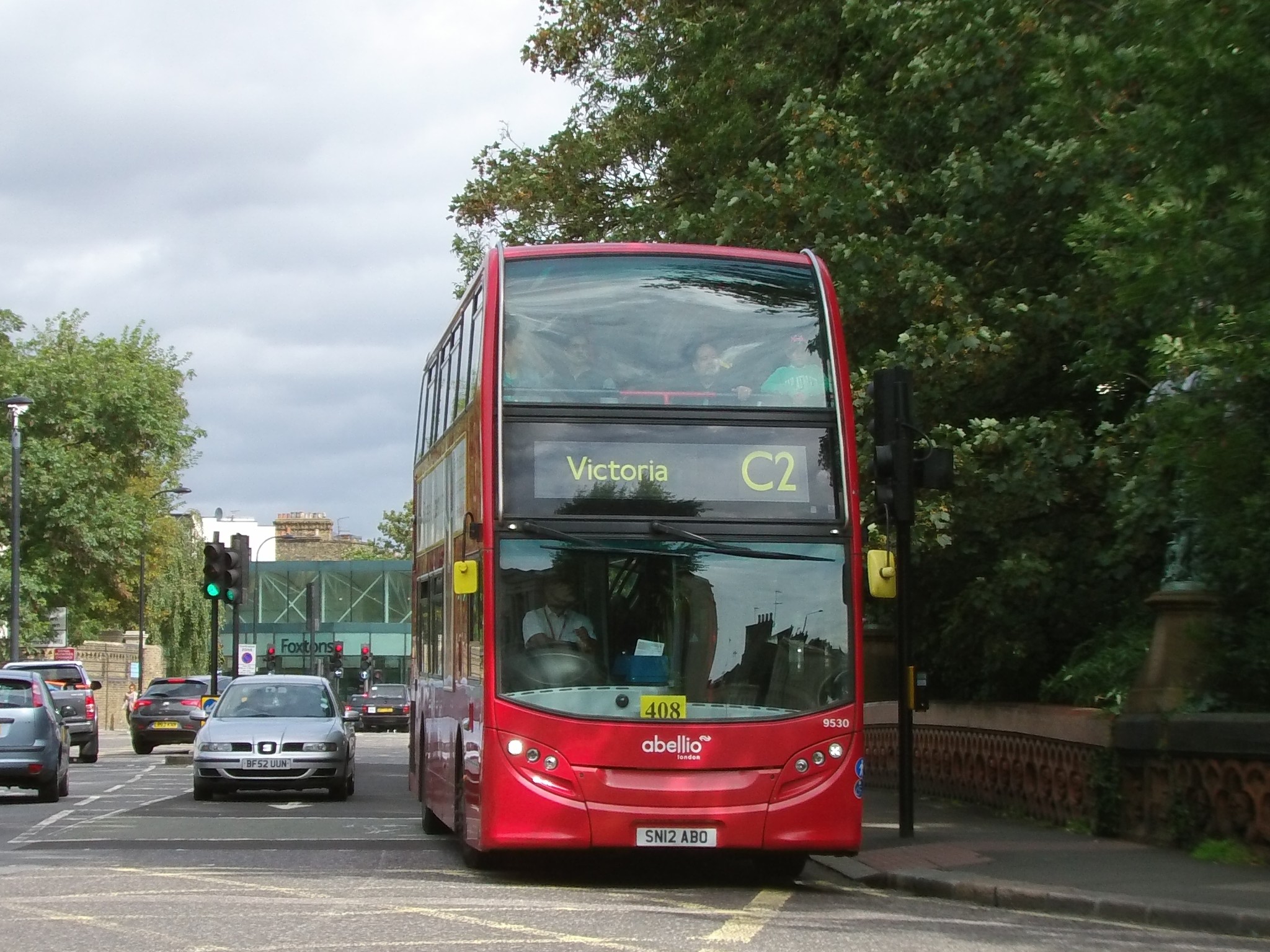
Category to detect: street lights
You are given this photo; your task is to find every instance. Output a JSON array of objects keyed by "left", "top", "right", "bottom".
[
  {"left": 2, "top": 396, "right": 34, "bottom": 658},
  {"left": 137, "top": 488, "right": 193, "bottom": 701},
  {"left": 801, "top": 609, "right": 824, "bottom": 636},
  {"left": 253, "top": 534, "right": 296, "bottom": 644}
]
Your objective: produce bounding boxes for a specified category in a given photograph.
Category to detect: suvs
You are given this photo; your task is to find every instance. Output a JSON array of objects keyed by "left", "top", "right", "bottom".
[
  {"left": 130, "top": 675, "right": 234, "bottom": 754},
  {"left": 362, "top": 683, "right": 411, "bottom": 733},
  {"left": 2, "top": 661, "right": 101, "bottom": 763}
]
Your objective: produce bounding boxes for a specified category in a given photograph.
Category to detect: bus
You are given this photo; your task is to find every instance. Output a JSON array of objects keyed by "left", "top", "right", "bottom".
[{"left": 406, "top": 245, "right": 895, "bottom": 884}]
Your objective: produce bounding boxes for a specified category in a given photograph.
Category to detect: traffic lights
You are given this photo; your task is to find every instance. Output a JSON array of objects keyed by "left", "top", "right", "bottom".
[
  {"left": 333, "top": 641, "right": 344, "bottom": 669},
  {"left": 871, "top": 443, "right": 896, "bottom": 526},
  {"left": 223, "top": 534, "right": 248, "bottom": 605},
  {"left": 360, "top": 644, "right": 371, "bottom": 670},
  {"left": 266, "top": 648, "right": 276, "bottom": 671},
  {"left": 329, "top": 656, "right": 337, "bottom": 672},
  {"left": 203, "top": 541, "right": 227, "bottom": 600}
]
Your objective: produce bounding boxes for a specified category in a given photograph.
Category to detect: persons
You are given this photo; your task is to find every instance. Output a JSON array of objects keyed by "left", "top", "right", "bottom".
[
  {"left": 523, "top": 572, "right": 604, "bottom": 667},
  {"left": 501, "top": 324, "right": 831, "bottom": 409},
  {"left": 123, "top": 683, "right": 138, "bottom": 733}
]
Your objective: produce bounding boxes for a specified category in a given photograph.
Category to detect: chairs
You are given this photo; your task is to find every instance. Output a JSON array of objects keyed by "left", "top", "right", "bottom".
[{"left": 8, "top": 688, "right": 25, "bottom": 705}]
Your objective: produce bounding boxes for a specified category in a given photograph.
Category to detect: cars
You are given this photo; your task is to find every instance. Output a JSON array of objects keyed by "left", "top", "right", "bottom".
[
  {"left": 189, "top": 673, "right": 361, "bottom": 803},
  {"left": 0, "top": 669, "right": 79, "bottom": 803},
  {"left": 344, "top": 694, "right": 363, "bottom": 721}
]
